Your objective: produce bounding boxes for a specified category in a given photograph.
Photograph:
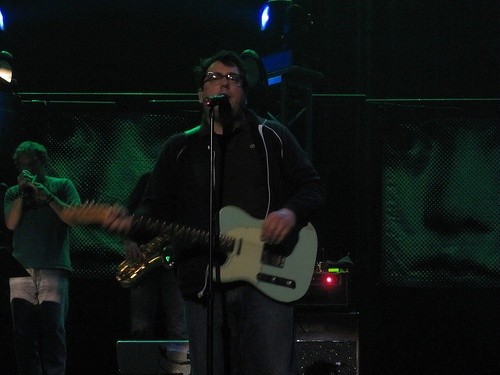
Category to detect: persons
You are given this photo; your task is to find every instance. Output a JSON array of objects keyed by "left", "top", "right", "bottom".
[
  {"left": 124, "top": 171, "right": 190, "bottom": 340},
  {"left": 17, "top": 102, "right": 202, "bottom": 274},
  {"left": 5, "top": 142, "right": 85, "bottom": 373},
  {"left": 102, "top": 55, "right": 325, "bottom": 372},
  {"left": 383, "top": 113, "right": 499, "bottom": 290}
]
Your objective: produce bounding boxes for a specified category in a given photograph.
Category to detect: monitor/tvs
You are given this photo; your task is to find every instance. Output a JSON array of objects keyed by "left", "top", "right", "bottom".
[{"left": 115, "top": 339, "right": 191, "bottom": 375}]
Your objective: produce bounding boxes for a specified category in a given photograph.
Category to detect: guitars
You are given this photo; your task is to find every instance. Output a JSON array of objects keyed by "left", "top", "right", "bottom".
[{"left": 56, "top": 197, "right": 319, "bottom": 304}]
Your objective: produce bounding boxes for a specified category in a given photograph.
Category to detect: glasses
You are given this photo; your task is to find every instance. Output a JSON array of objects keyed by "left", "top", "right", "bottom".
[{"left": 203, "top": 73, "right": 244, "bottom": 83}]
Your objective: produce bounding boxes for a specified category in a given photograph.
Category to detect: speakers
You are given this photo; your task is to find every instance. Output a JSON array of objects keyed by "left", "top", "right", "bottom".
[
  {"left": 294, "top": 271, "right": 349, "bottom": 306},
  {"left": 294, "top": 320, "right": 359, "bottom": 375}
]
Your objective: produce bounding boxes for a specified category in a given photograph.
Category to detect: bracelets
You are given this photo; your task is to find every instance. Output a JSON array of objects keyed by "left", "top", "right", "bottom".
[{"left": 43, "top": 192, "right": 55, "bottom": 204}]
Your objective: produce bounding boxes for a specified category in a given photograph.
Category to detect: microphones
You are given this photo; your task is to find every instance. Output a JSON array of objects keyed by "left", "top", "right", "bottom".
[
  {"left": 203, "top": 94, "right": 229, "bottom": 106},
  {"left": 25, "top": 178, "right": 31, "bottom": 183}
]
